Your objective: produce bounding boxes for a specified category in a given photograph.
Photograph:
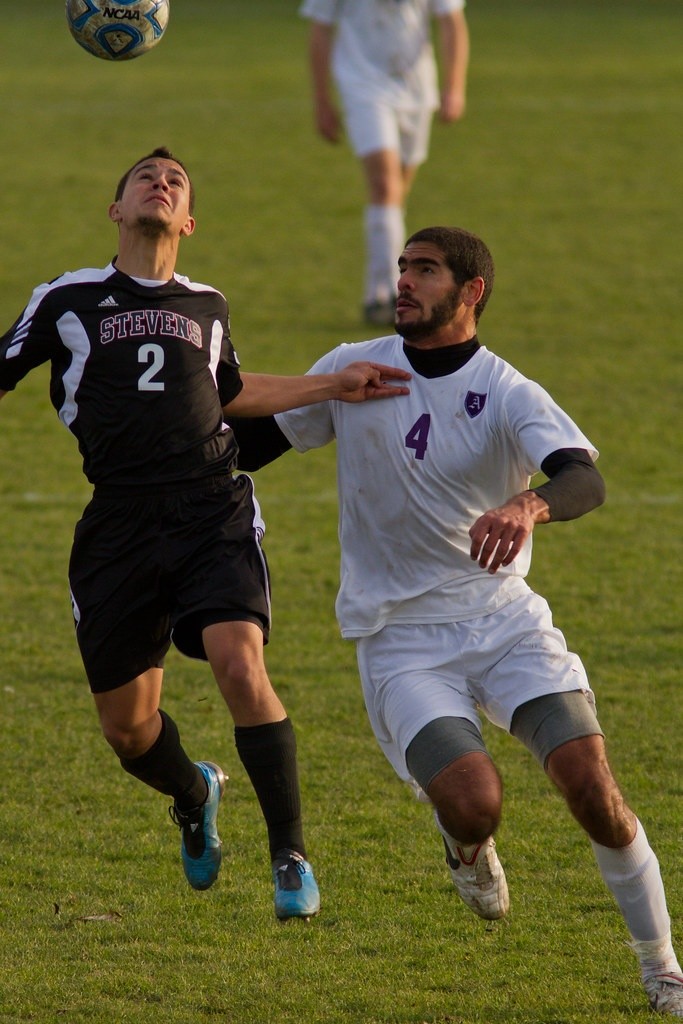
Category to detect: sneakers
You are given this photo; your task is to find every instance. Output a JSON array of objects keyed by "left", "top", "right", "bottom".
[
  {"left": 167, "top": 761, "right": 234, "bottom": 893},
  {"left": 642, "top": 971, "right": 683, "bottom": 1017},
  {"left": 271, "top": 850, "right": 320, "bottom": 925},
  {"left": 437, "top": 815, "right": 510, "bottom": 920}
]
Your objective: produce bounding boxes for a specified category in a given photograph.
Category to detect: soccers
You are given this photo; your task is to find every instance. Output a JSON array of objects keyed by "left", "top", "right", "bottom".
[{"left": 65, "top": 0, "right": 172, "bottom": 63}]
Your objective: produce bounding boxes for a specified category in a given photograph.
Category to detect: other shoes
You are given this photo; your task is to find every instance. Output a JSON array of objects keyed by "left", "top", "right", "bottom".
[{"left": 364, "top": 296, "right": 400, "bottom": 326}]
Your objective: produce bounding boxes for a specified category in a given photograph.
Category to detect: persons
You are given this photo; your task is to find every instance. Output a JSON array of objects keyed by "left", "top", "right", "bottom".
[
  {"left": 0, "top": 142, "right": 414, "bottom": 920},
  {"left": 231, "top": 225, "right": 683, "bottom": 1018},
  {"left": 304, "top": 0, "right": 471, "bottom": 325}
]
